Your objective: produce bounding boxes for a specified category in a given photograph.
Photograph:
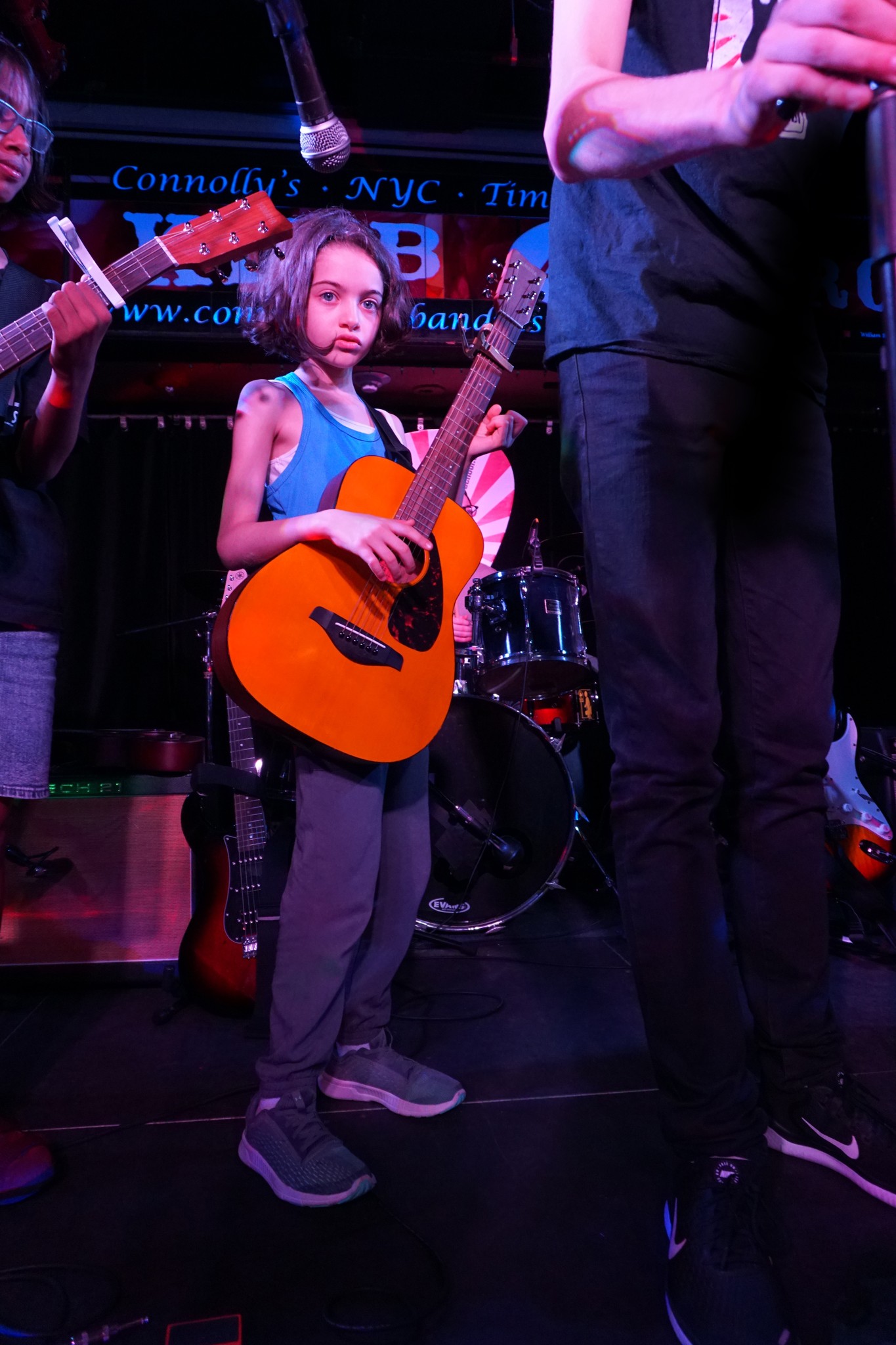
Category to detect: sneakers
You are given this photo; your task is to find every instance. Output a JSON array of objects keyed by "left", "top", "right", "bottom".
[
  {"left": 315, "top": 1025, "right": 465, "bottom": 1118},
  {"left": 239, "top": 1086, "right": 376, "bottom": 1209},
  {"left": 663, "top": 1148, "right": 789, "bottom": 1345},
  {"left": 764, "top": 1068, "right": 896, "bottom": 1210}
]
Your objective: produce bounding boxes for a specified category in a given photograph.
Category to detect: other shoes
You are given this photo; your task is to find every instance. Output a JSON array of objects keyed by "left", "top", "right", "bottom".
[{"left": 0, "top": 1118, "right": 52, "bottom": 1205}]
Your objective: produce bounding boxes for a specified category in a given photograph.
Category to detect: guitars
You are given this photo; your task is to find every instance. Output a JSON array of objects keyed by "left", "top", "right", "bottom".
[
  {"left": 208, "top": 248, "right": 549, "bottom": 768},
  {"left": 0, "top": 189, "right": 294, "bottom": 377},
  {"left": 824, "top": 713, "right": 893, "bottom": 881},
  {"left": 176, "top": 691, "right": 288, "bottom": 1001}
]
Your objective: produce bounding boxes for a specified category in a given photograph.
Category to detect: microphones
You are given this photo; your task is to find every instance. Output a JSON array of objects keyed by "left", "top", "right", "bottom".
[
  {"left": 499, "top": 843, "right": 521, "bottom": 861},
  {"left": 263, "top": 0, "right": 352, "bottom": 172},
  {"left": 522, "top": 519, "right": 539, "bottom": 564},
  {"left": 25, "top": 858, "right": 73, "bottom": 879}
]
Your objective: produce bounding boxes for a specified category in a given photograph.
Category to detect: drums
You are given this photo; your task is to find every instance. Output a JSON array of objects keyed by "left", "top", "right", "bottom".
[
  {"left": 464, "top": 563, "right": 597, "bottom": 696},
  {"left": 406, "top": 693, "right": 577, "bottom": 932}
]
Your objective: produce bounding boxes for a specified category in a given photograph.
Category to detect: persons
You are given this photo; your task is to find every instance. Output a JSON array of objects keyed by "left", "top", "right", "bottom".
[
  {"left": 0, "top": 49, "right": 113, "bottom": 911},
  {"left": 215, "top": 205, "right": 526, "bottom": 1210},
  {"left": 543, "top": 0, "right": 896, "bottom": 1345}
]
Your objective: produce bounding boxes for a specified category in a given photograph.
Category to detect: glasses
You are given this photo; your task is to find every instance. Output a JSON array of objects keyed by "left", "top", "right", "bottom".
[{"left": 0, "top": 97, "right": 55, "bottom": 158}]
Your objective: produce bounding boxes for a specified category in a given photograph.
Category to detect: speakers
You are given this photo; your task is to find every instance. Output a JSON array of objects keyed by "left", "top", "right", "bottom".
[{"left": 0, "top": 772, "right": 199, "bottom": 973}]
